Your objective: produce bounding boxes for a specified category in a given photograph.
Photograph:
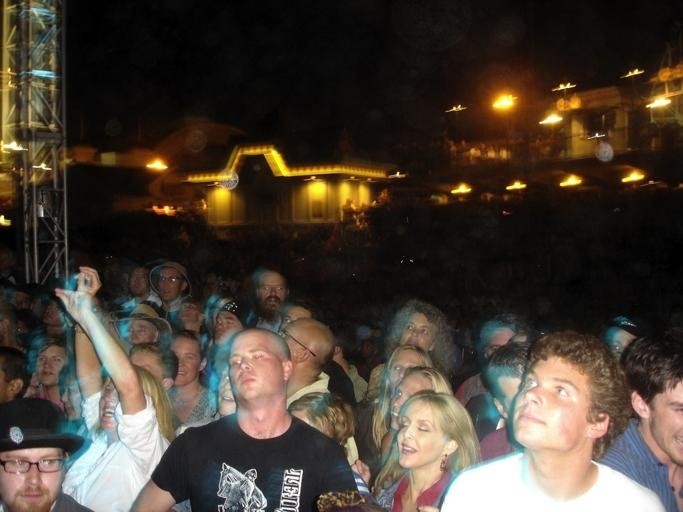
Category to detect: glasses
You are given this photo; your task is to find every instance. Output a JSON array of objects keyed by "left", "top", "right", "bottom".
[
  {"left": 0, "top": 452, "right": 68, "bottom": 474},
  {"left": 279, "top": 328, "right": 317, "bottom": 359},
  {"left": 158, "top": 274, "right": 180, "bottom": 284}
]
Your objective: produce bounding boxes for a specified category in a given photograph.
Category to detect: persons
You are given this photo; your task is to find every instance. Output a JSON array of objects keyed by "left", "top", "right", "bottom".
[{"left": 1, "top": 204, "right": 683, "bottom": 512}]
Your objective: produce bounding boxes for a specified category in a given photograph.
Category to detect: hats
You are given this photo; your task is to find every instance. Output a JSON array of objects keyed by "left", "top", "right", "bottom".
[
  {"left": 149, "top": 262, "right": 193, "bottom": 296},
  {"left": 115, "top": 302, "right": 174, "bottom": 352},
  {"left": 218, "top": 300, "right": 242, "bottom": 323},
  {"left": 0, "top": 397, "right": 84, "bottom": 453}
]
